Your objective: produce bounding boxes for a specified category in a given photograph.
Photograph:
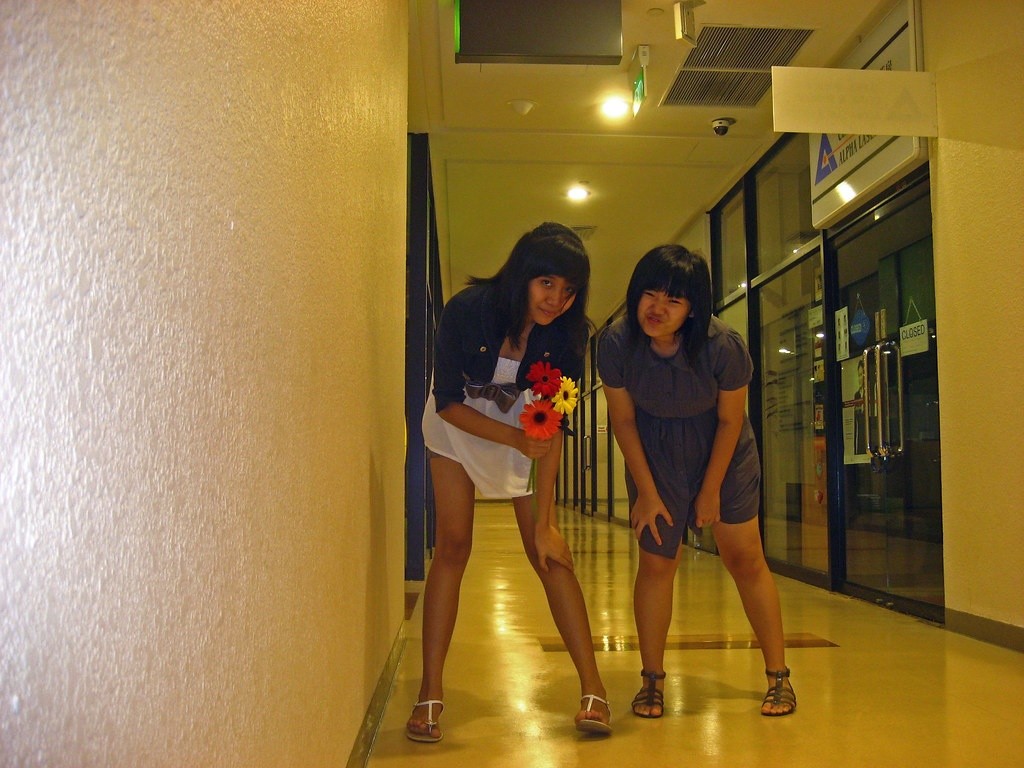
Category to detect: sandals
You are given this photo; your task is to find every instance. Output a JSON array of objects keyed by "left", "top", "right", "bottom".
[
  {"left": 407, "top": 700, "right": 444, "bottom": 741},
  {"left": 632, "top": 669, "right": 666, "bottom": 718},
  {"left": 761, "top": 666, "right": 796, "bottom": 715},
  {"left": 576, "top": 695, "right": 613, "bottom": 732}
]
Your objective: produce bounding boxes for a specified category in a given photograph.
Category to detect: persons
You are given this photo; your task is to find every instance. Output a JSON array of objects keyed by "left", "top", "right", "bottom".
[
  {"left": 595, "top": 244, "right": 801, "bottom": 715},
  {"left": 400, "top": 221, "right": 612, "bottom": 744},
  {"left": 852, "top": 361, "right": 872, "bottom": 456}
]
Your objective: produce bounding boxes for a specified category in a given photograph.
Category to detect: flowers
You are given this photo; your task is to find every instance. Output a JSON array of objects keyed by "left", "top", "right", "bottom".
[{"left": 519, "top": 360, "right": 578, "bottom": 516}]
